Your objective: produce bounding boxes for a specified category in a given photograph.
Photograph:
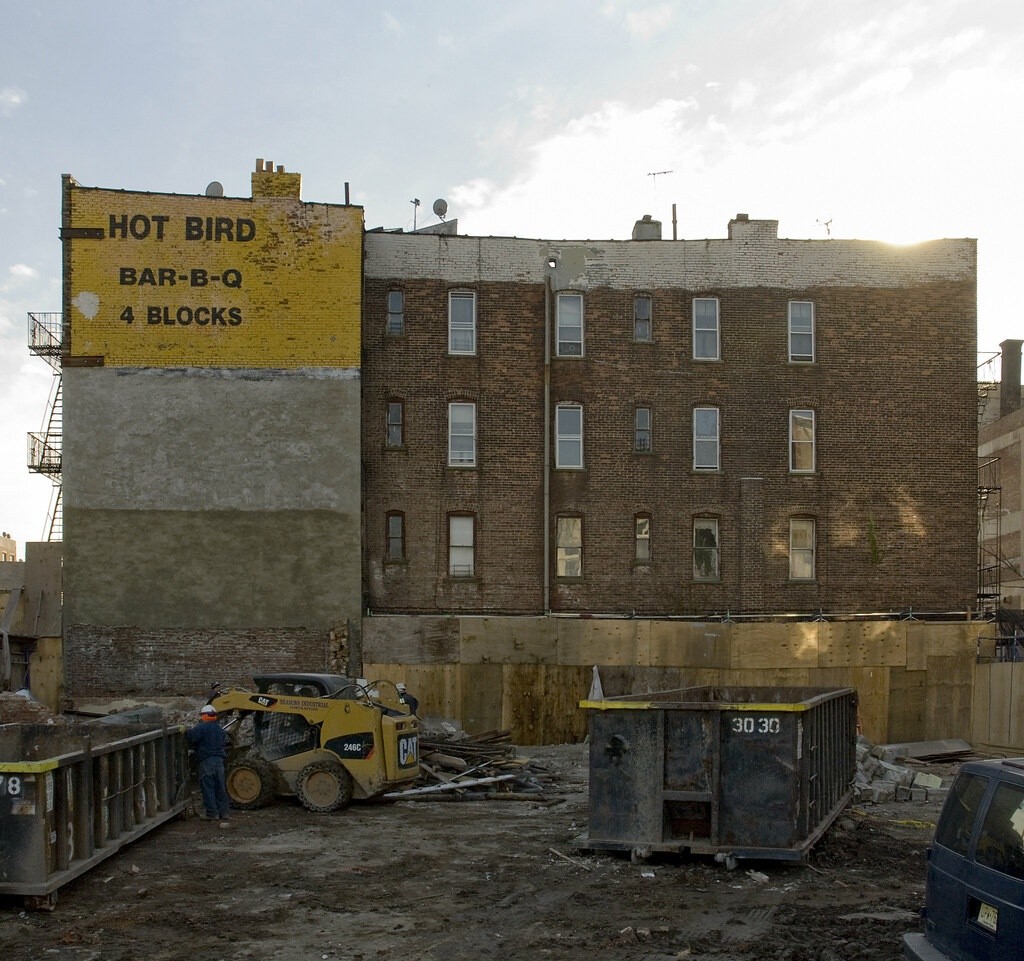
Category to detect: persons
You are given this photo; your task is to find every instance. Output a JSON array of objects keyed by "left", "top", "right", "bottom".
[
  {"left": 396, "top": 683, "right": 419, "bottom": 716},
  {"left": 185, "top": 704, "right": 230, "bottom": 820}
]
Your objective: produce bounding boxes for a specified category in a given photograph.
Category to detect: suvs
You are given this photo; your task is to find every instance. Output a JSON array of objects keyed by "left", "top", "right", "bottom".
[{"left": 900, "top": 754, "right": 1023, "bottom": 961}]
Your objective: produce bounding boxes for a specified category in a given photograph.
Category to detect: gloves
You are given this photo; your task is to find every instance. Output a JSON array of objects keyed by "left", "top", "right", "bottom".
[{"left": 179, "top": 725, "right": 185, "bottom": 734}]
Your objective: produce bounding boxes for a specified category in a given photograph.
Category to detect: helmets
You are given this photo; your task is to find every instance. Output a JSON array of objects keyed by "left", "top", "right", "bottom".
[
  {"left": 396, "top": 683, "right": 405, "bottom": 692},
  {"left": 199, "top": 705, "right": 218, "bottom": 714}
]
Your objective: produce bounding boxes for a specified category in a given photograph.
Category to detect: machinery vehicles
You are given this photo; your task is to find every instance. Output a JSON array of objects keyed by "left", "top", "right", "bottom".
[{"left": 210, "top": 670, "right": 420, "bottom": 814}]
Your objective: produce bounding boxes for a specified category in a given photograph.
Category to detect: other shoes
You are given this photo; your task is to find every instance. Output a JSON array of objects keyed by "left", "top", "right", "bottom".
[
  {"left": 219, "top": 814, "right": 229, "bottom": 820},
  {"left": 200, "top": 815, "right": 219, "bottom": 821}
]
